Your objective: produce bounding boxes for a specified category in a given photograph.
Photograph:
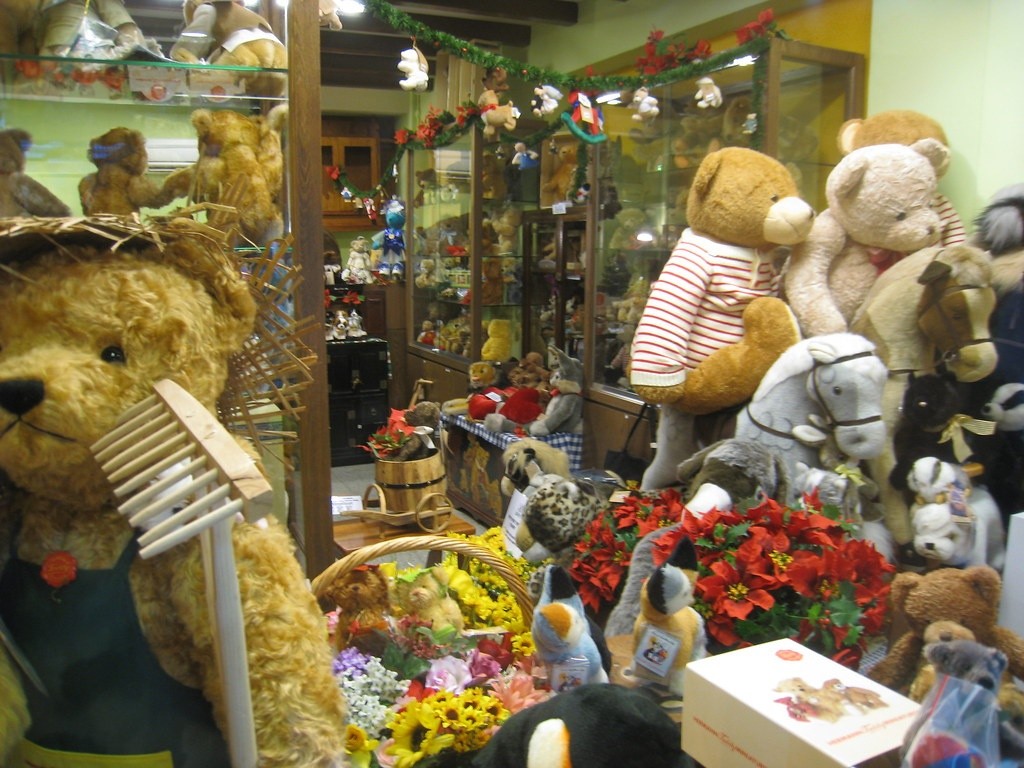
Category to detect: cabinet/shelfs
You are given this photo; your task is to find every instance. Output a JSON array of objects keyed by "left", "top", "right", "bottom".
[
  {"left": 322, "top": 114, "right": 403, "bottom": 230},
  {"left": 402, "top": 121, "right": 550, "bottom": 414},
  {"left": 583, "top": 39, "right": 866, "bottom": 482},
  {"left": 515, "top": 207, "right": 588, "bottom": 372},
  {"left": 326, "top": 336, "right": 391, "bottom": 468},
  {"left": 0, "top": 0, "right": 334, "bottom": 581}
]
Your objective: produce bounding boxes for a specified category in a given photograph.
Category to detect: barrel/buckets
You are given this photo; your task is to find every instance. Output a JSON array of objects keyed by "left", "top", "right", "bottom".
[{"left": 374, "top": 447, "right": 445, "bottom": 511}]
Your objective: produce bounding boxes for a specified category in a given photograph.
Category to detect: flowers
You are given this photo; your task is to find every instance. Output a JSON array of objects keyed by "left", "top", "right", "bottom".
[
  {"left": 322, "top": 526, "right": 555, "bottom": 768},
  {"left": 353, "top": 404, "right": 417, "bottom": 460},
  {"left": 567, "top": 487, "right": 686, "bottom": 616},
  {"left": 326, "top": 7, "right": 787, "bottom": 202},
  {"left": 640, "top": 483, "right": 896, "bottom": 673}
]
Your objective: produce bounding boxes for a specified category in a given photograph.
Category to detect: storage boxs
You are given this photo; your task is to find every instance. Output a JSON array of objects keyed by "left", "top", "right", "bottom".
[{"left": 680, "top": 637, "right": 922, "bottom": 768}]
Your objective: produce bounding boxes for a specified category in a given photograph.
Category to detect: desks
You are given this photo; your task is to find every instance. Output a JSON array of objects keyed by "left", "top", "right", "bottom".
[
  {"left": 440, "top": 411, "right": 584, "bottom": 528},
  {"left": 334, "top": 515, "right": 476, "bottom": 575}
]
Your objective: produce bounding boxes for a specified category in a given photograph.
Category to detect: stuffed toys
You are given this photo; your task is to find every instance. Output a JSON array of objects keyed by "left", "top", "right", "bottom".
[
  {"left": 442, "top": 344, "right": 584, "bottom": 435},
  {"left": 323, "top": 198, "right": 405, "bottom": 343},
  {"left": 501, "top": 76, "right": 1024, "bottom": 712},
  {"left": 0, "top": 106, "right": 288, "bottom": 247},
  {"left": 382, "top": 402, "right": 441, "bottom": 463},
  {"left": 397, "top": 46, "right": 429, "bottom": 92},
  {"left": 0, "top": 0, "right": 342, "bottom": 97},
  {"left": 414, "top": 156, "right": 522, "bottom": 361},
  {"left": 774, "top": 678, "right": 890, "bottom": 722},
  {"left": 477, "top": 66, "right": 521, "bottom": 134},
  {"left": 325, "top": 564, "right": 610, "bottom": 690},
  {"left": 900, "top": 640, "right": 1024, "bottom": 768},
  {"left": 0, "top": 202, "right": 345, "bottom": 768},
  {"left": 413, "top": 683, "right": 696, "bottom": 768}
]
